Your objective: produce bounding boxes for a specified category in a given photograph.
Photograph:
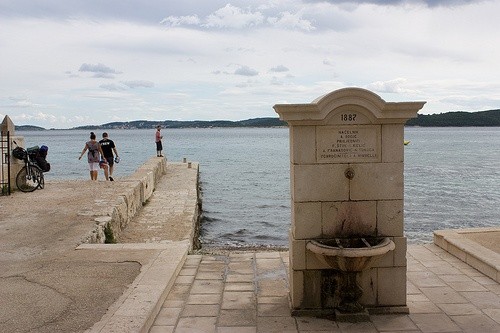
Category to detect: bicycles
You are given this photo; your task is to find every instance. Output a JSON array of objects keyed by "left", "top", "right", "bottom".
[{"left": 12, "top": 140, "right": 51, "bottom": 192}]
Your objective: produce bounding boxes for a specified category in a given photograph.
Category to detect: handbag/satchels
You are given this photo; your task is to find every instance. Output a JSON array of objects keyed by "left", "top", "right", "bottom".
[{"left": 12, "top": 145, "right": 51, "bottom": 173}]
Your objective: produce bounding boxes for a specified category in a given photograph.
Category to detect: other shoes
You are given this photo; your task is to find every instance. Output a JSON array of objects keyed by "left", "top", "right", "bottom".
[{"left": 109, "top": 177, "right": 114, "bottom": 181}]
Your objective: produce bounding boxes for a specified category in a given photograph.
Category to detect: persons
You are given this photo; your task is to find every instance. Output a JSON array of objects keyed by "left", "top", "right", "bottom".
[
  {"left": 155, "top": 125, "right": 163, "bottom": 157},
  {"left": 79, "top": 132, "right": 107, "bottom": 180},
  {"left": 98, "top": 133, "right": 120, "bottom": 181}
]
